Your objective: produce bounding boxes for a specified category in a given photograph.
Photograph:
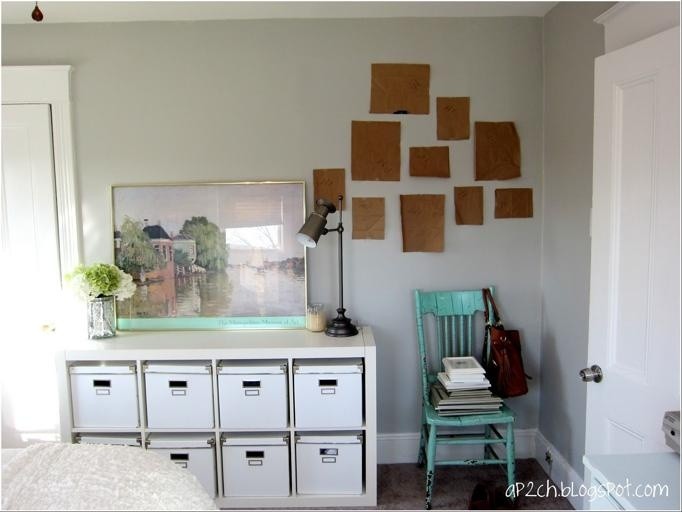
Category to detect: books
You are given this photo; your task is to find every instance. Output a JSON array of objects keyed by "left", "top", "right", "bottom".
[{"left": 431, "top": 355, "right": 504, "bottom": 416}]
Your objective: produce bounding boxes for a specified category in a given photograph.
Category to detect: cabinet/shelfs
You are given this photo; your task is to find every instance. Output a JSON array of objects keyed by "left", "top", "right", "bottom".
[{"left": 57, "top": 326, "right": 377, "bottom": 508}]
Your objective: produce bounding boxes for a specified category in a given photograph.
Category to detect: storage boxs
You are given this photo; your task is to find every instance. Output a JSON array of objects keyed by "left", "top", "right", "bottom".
[
  {"left": 293, "top": 359, "right": 363, "bottom": 426},
  {"left": 69, "top": 361, "right": 140, "bottom": 428},
  {"left": 217, "top": 361, "right": 288, "bottom": 428},
  {"left": 75, "top": 433, "right": 141, "bottom": 448},
  {"left": 146, "top": 433, "right": 216, "bottom": 499},
  {"left": 296, "top": 432, "right": 363, "bottom": 495},
  {"left": 220, "top": 432, "right": 290, "bottom": 496},
  {"left": 142, "top": 360, "right": 215, "bottom": 429}
]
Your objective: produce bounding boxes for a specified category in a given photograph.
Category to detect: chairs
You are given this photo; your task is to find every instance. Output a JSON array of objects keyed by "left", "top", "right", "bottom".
[
  {"left": 415, "top": 286, "right": 517, "bottom": 509},
  {"left": 1, "top": 442, "right": 218, "bottom": 511}
]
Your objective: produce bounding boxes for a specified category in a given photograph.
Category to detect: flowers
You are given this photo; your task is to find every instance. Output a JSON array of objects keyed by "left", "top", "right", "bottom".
[{"left": 63, "top": 262, "right": 137, "bottom": 301}]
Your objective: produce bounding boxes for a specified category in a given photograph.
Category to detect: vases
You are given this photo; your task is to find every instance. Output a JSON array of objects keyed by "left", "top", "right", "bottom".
[{"left": 87, "top": 294, "right": 116, "bottom": 339}]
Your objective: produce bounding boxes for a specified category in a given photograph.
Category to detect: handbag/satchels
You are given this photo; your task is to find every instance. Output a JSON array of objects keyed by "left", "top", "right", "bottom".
[{"left": 478, "top": 285, "right": 533, "bottom": 401}]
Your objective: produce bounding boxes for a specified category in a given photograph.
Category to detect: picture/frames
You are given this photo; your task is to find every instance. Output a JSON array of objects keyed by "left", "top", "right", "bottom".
[{"left": 109, "top": 181, "right": 308, "bottom": 331}]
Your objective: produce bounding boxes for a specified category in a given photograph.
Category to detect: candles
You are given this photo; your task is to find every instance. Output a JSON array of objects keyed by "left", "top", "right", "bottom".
[{"left": 310, "top": 311, "right": 324, "bottom": 331}]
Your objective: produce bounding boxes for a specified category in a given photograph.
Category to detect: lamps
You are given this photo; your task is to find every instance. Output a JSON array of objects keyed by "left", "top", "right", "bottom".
[{"left": 297, "top": 195, "right": 359, "bottom": 337}]
